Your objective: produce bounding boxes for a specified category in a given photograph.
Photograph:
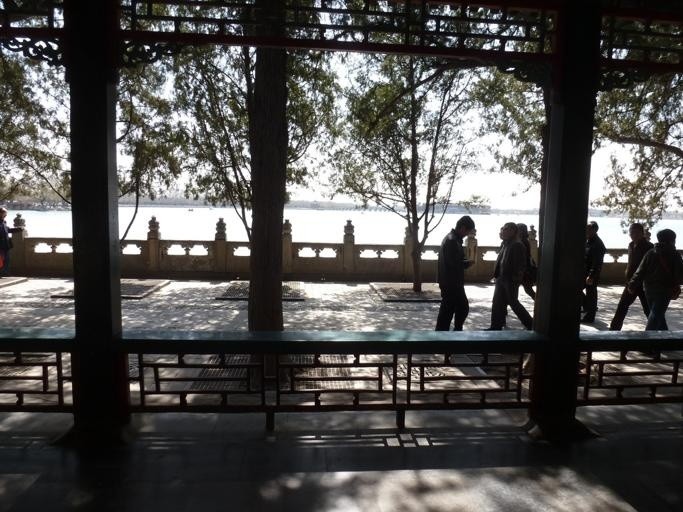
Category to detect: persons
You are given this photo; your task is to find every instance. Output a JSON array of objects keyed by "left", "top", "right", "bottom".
[
  {"left": 515, "top": 223, "right": 535, "bottom": 300},
  {"left": 483, "top": 222, "right": 532, "bottom": 330},
  {"left": 433, "top": 215, "right": 475, "bottom": 330},
  {"left": 581, "top": 221, "right": 603, "bottom": 322},
  {"left": 0, "top": 207, "right": 23, "bottom": 276},
  {"left": 609, "top": 223, "right": 669, "bottom": 330},
  {"left": 579, "top": 292, "right": 599, "bottom": 314},
  {"left": 626, "top": 228, "right": 682, "bottom": 356}
]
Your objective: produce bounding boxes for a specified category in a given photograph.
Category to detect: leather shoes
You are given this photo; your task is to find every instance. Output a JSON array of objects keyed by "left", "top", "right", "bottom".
[
  {"left": 483, "top": 328, "right": 503, "bottom": 330},
  {"left": 580, "top": 318, "right": 593, "bottom": 323}
]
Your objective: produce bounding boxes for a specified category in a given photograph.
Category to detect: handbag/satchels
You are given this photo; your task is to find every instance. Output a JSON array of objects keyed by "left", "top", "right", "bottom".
[
  {"left": 671, "top": 281, "right": 681, "bottom": 300},
  {"left": 530, "top": 265, "right": 538, "bottom": 283}
]
